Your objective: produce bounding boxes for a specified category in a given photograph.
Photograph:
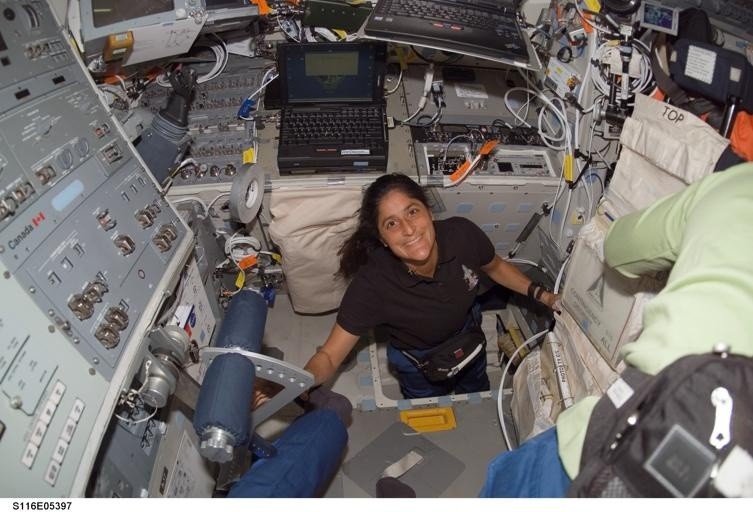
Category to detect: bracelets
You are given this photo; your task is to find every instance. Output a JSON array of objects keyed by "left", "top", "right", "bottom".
[
  {"left": 534, "top": 285, "right": 552, "bottom": 306},
  {"left": 526, "top": 279, "right": 541, "bottom": 303}
]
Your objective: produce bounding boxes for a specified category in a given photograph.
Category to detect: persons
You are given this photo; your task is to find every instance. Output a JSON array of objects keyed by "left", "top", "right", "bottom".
[
  {"left": 475, "top": 155, "right": 752, "bottom": 497},
  {"left": 221, "top": 383, "right": 354, "bottom": 499},
  {"left": 243, "top": 170, "right": 560, "bottom": 413},
  {"left": 663, "top": 6, "right": 719, "bottom": 48}
]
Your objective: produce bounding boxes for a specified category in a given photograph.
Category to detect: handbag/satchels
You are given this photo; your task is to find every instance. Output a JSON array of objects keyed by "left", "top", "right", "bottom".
[
  {"left": 567, "top": 351, "right": 753, "bottom": 497},
  {"left": 416, "top": 320, "right": 488, "bottom": 383}
]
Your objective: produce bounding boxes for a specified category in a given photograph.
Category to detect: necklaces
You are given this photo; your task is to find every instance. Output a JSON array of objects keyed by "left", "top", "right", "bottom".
[{"left": 406, "top": 261, "right": 420, "bottom": 276}]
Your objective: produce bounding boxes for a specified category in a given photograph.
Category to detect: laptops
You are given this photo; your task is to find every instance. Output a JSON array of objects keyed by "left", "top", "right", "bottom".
[
  {"left": 277, "top": 42, "right": 389, "bottom": 176},
  {"left": 363, "top": 0, "right": 530, "bottom": 63}
]
